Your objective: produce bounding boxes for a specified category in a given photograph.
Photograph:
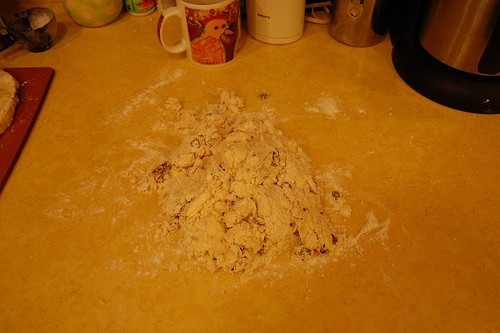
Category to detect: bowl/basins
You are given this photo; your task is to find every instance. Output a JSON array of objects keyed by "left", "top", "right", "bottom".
[{"left": 5, "top": 7, "right": 58, "bottom": 52}]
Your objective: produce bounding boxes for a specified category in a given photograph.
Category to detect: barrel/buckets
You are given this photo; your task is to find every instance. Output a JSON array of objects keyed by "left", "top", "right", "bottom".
[{"left": 392, "top": 0, "right": 499, "bottom": 115}]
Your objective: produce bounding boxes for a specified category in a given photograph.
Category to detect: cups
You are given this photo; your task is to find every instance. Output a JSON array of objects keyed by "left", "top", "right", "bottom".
[
  {"left": 328, "top": 0, "right": 390, "bottom": 46},
  {"left": 126, "top": 0, "right": 155, "bottom": 16},
  {"left": 59, "top": 0, "right": 123, "bottom": 26},
  {"left": 246, "top": 0, "right": 306, "bottom": 43},
  {"left": 157, "top": 0, "right": 241, "bottom": 66}
]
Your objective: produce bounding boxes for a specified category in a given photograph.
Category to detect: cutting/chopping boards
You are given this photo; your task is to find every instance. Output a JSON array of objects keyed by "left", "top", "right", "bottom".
[{"left": 1, "top": 66, "right": 55, "bottom": 191}]
